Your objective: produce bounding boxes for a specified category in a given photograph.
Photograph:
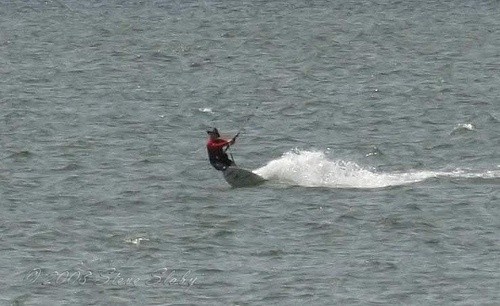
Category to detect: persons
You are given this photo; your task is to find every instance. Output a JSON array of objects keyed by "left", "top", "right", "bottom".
[{"left": 206, "top": 128, "right": 236, "bottom": 172}]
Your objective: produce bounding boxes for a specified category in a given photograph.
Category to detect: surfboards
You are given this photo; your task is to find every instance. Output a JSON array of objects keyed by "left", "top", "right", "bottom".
[{"left": 223, "top": 166, "right": 265, "bottom": 188}]
